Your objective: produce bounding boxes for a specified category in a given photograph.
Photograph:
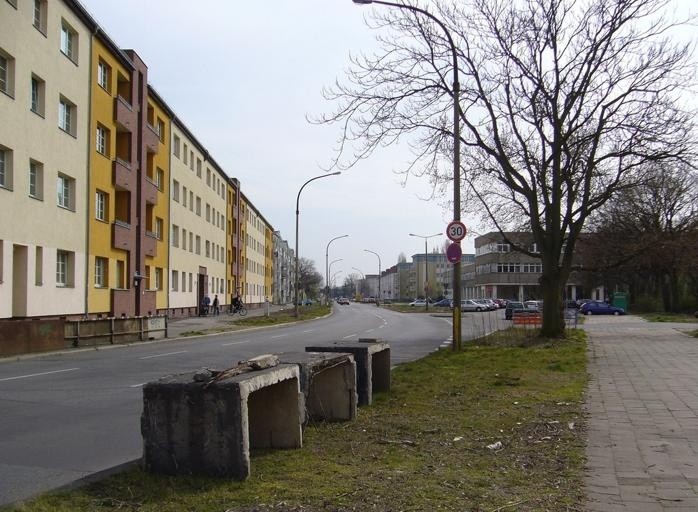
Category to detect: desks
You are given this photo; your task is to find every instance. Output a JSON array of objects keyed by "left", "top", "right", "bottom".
[{"left": 141, "top": 339, "right": 392, "bottom": 478}]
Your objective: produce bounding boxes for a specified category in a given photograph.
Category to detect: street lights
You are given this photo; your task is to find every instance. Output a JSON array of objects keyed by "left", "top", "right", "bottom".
[
  {"left": 325, "top": 234, "right": 348, "bottom": 305},
  {"left": 295, "top": 171, "right": 341, "bottom": 318},
  {"left": 363, "top": 249, "right": 381, "bottom": 301},
  {"left": 352, "top": 0, "right": 461, "bottom": 351},
  {"left": 409, "top": 232, "right": 443, "bottom": 311}
]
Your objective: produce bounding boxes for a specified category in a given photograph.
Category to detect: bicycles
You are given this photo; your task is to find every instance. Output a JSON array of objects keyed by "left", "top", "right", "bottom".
[{"left": 226, "top": 304, "right": 247, "bottom": 316}]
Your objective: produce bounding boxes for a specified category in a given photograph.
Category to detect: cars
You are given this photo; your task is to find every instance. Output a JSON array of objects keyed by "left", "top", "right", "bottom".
[
  {"left": 341, "top": 298, "right": 349, "bottom": 304},
  {"left": 294, "top": 299, "right": 312, "bottom": 306},
  {"left": 451, "top": 299, "right": 543, "bottom": 320},
  {"left": 577, "top": 299, "right": 624, "bottom": 315},
  {"left": 409, "top": 299, "right": 452, "bottom": 307}
]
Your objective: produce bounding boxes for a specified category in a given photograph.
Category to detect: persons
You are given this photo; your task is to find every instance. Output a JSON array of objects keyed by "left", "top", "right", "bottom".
[
  {"left": 231, "top": 295, "right": 244, "bottom": 313},
  {"left": 211, "top": 294, "right": 222, "bottom": 316}
]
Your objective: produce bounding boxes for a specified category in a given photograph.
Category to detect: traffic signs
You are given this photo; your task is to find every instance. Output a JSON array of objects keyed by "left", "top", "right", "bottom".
[{"left": 446, "top": 222, "right": 466, "bottom": 242}]
